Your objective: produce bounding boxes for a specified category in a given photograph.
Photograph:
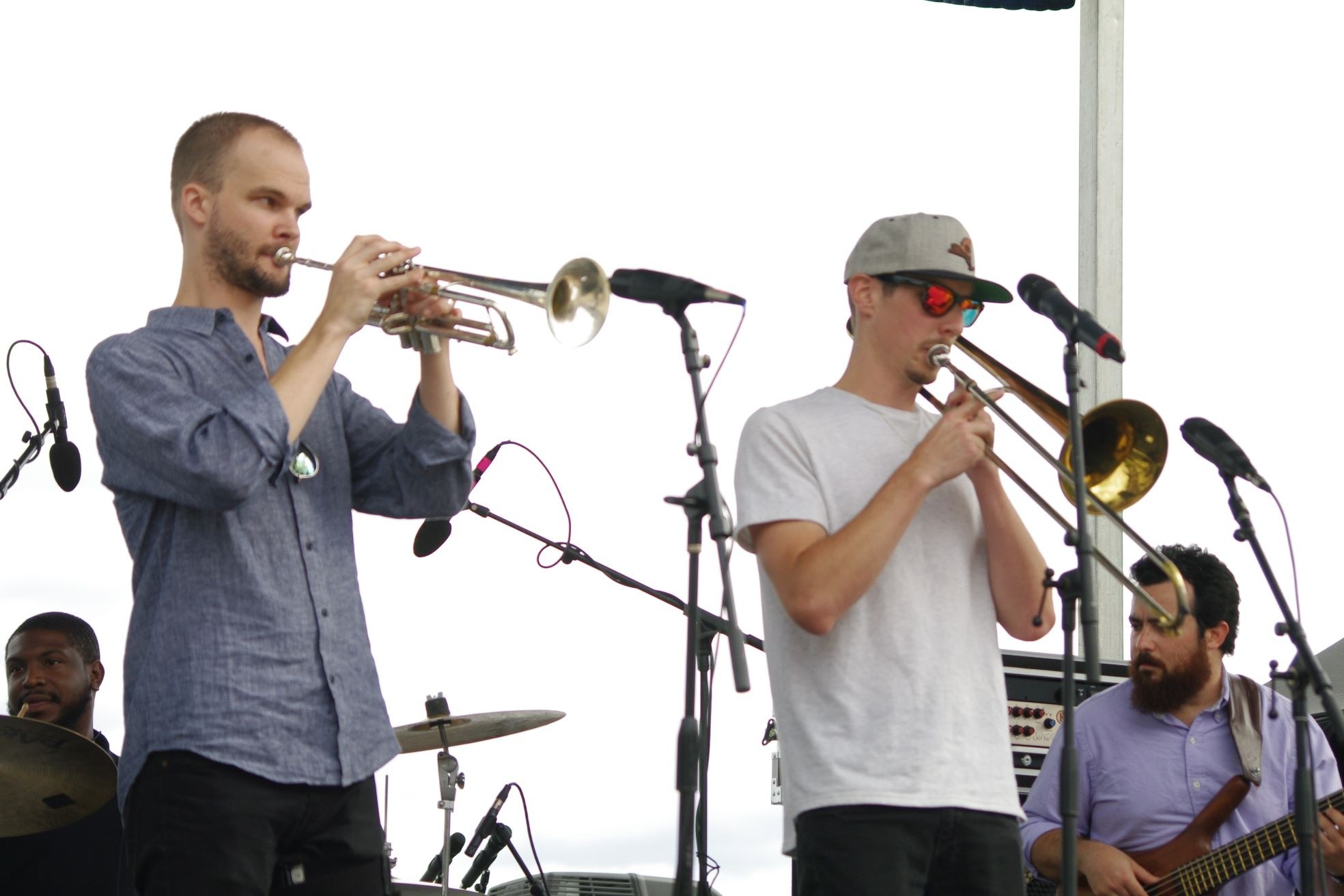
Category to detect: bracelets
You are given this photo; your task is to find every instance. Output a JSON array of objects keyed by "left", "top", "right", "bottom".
[{"left": 1325, "top": 871, "right": 1344, "bottom": 886}]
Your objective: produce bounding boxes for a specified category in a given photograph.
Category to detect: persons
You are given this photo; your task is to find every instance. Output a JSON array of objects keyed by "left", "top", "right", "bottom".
[
  {"left": 732, "top": 212, "right": 1055, "bottom": 896},
  {"left": 85, "top": 112, "right": 478, "bottom": 896},
  {"left": 1019, "top": 546, "right": 1344, "bottom": 896},
  {"left": 0, "top": 611, "right": 121, "bottom": 896}
]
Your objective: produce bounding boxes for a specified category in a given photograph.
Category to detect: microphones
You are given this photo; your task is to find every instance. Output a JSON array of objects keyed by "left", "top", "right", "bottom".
[
  {"left": 1016, "top": 274, "right": 1123, "bottom": 361},
  {"left": 462, "top": 825, "right": 514, "bottom": 887},
  {"left": 608, "top": 269, "right": 744, "bottom": 307},
  {"left": 421, "top": 832, "right": 466, "bottom": 882},
  {"left": 464, "top": 784, "right": 511, "bottom": 857},
  {"left": 44, "top": 356, "right": 81, "bottom": 491},
  {"left": 1180, "top": 417, "right": 1271, "bottom": 493},
  {"left": 414, "top": 444, "right": 499, "bottom": 558}
]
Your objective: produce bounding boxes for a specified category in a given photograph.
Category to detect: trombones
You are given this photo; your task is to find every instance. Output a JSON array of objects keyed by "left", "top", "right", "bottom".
[{"left": 846, "top": 313, "right": 1191, "bottom": 634}]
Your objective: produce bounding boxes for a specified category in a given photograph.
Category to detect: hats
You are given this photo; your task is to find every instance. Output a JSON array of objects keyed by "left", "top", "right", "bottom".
[{"left": 843, "top": 212, "right": 1014, "bottom": 304}]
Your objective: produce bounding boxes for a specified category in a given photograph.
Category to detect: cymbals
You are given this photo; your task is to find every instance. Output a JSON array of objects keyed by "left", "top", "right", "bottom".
[
  {"left": 390, "top": 881, "right": 489, "bottom": 896},
  {"left": 393, "top": 708, "right": 566, "bottom": 753},
  {"left": 0, "top": 714, "right": 118, "bottom": 838}
]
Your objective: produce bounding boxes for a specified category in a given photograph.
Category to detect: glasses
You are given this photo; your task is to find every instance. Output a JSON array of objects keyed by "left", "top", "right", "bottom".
[
  {"left": 880, "top": 276, "right": 985, "bottom": 328},
  {"left": 289, "top": 440, "right": 319, "bottom": 480}
]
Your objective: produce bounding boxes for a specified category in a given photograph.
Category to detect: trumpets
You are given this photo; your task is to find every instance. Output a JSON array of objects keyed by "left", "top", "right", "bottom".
[{"left": 272, "top": 245, "right": 610, "bottom": 356}]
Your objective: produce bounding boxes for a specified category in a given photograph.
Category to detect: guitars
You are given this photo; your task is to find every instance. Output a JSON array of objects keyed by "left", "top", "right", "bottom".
[{"left": 1027, "top": 771, "right": 1344, "bottom": 896}]
